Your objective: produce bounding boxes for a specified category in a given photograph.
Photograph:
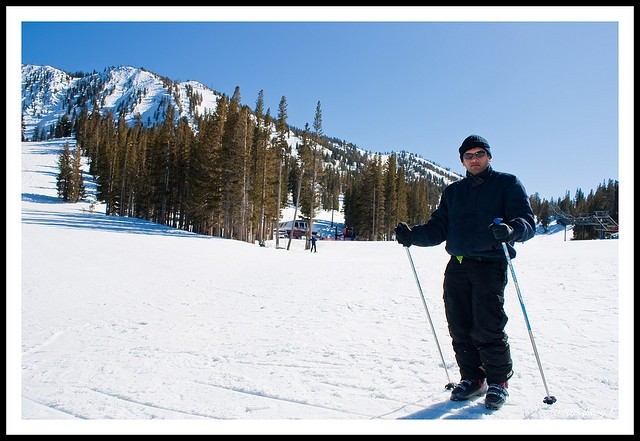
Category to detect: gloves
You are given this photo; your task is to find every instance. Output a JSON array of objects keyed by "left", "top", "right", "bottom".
[
  {"left": 395, "top": 222, "right": 412, "bottom": 248},
  {"left": 488, "top": 222, "right": 514, "bottom": 241}
]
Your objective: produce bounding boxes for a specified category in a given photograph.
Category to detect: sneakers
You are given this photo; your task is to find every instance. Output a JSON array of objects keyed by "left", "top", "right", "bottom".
[
  {"left": 453, "top": 378, "right": 488, "bottom": 401},
  {"left": 485, "top": 384, "right": 509, "bottom": 410}
]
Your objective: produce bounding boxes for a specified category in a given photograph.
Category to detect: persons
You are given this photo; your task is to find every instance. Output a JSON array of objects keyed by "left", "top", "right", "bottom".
[
  {"left": 393, "top": 134, "right": 536, "bottom": 410},
  {"left": 309, "top": 236, "right": 318, "bottom": 253}
]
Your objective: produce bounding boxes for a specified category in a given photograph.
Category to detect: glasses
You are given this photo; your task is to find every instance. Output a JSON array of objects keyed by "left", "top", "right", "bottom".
[{"left": 463, "top": 150, "right": 486, "bottom": 159}]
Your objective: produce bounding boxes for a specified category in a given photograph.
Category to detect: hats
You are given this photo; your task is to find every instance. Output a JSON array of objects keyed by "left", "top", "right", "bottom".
[{"left": 459, "top": 135, "right": 492, "bottom": 163}]
